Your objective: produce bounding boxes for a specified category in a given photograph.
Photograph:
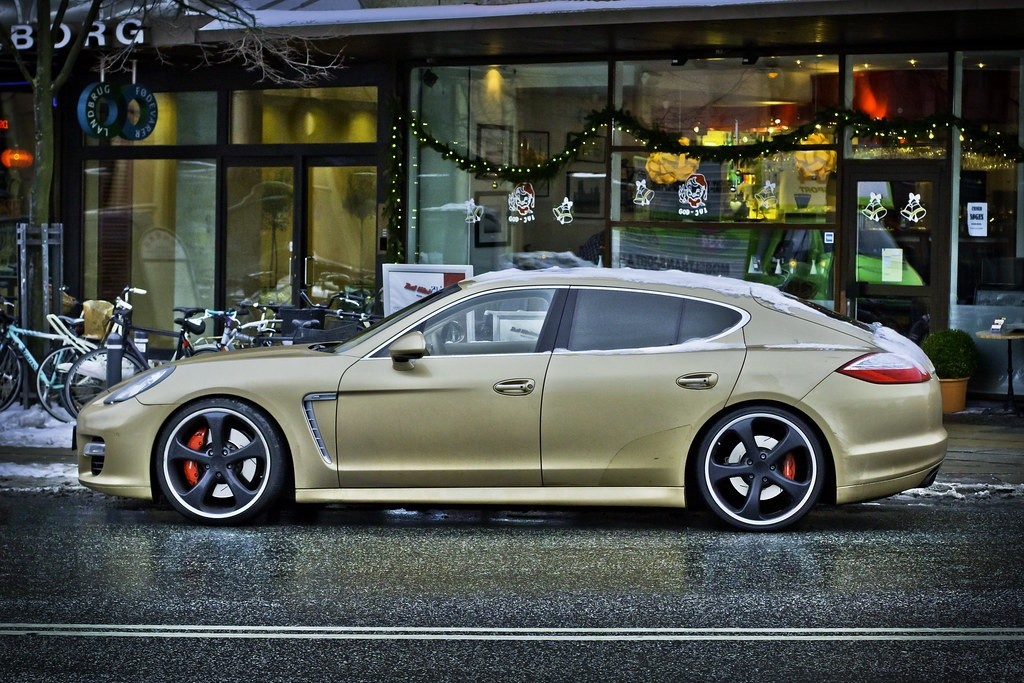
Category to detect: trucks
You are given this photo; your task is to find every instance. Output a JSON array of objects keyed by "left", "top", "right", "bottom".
[{"left": 623, "top": 201, "right": 930, "bottom": 343}]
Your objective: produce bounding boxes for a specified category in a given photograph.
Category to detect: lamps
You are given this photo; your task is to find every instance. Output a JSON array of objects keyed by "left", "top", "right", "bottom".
[
  {"left": 671, "top": 59, "right": 689, "bottom": 66},
  {"left": 421, "top": 68, "right": 439, "bottom": 87},
  {"left": 743, "top": 57, "right": 759, "bottom": 65}
]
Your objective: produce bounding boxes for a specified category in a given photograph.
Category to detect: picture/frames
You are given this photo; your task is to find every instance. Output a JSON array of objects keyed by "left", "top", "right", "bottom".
[
  {"left": 518, "top": 130, "right": 550, "bottom": 197},
  {"left": 477, "top": 123, "right": 513, "bottom": 179},
  {"left": 565, "top": 171, "right": 606, "bottom": 220},
  {"left": 568, "top": 132, "right": 606, "bottom": 163},
  {"left": 475, "top": 191, "right": 511, "bottom": 248}
]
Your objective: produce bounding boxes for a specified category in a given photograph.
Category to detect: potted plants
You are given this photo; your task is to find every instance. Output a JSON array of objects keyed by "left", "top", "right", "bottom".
[{"left": 924, "top": 327, "right": 976, "bottom": 413}]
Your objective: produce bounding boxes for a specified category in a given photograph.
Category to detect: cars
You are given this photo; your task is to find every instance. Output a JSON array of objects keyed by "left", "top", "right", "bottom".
[{"left": 74, "top": 267, "right": 950, "bottom": 532}]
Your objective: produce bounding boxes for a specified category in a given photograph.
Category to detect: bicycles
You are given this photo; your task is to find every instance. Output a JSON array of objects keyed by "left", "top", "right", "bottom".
[{"left": 0, "top": 286, "right": 381, "bottom": 424}]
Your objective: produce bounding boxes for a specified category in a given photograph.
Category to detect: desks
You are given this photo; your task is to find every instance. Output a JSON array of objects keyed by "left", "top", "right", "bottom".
[{"left": 975, "top": 328, "right": 1024, "bottom": 414}]
